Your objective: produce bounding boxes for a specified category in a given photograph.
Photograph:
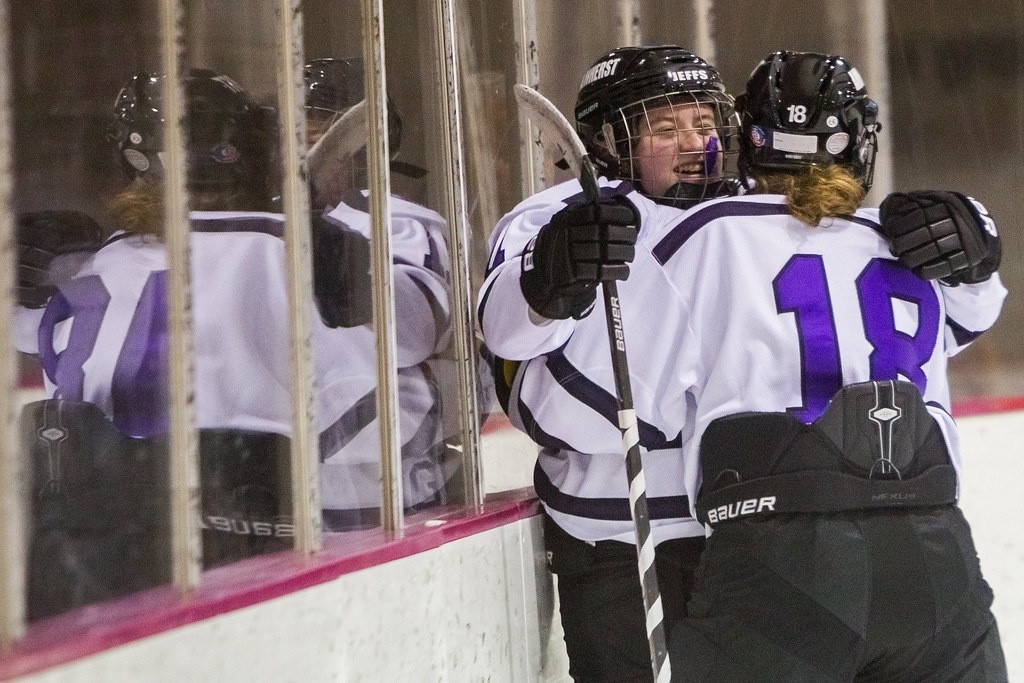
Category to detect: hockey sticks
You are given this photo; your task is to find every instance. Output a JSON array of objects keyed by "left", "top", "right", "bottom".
[
  {"left": 513, "top": 81, "right": 672, "bottom": 682},
  {"left": 304, "top": 98, "right": 371, "bottom": 192}
]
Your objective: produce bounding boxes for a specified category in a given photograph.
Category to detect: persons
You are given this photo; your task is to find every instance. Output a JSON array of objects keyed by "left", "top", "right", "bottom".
[
  {"left": 479, "top": 47, "right": 1010, "bottom": 683},
  {"left": 204, "top": 53, "right": 466, "bottom": 543},
  {"left": 485, "top": 47, "right": 1013, "bottom": 683},
  {"left": 36, "top": 63, "right": 457, "bottom": 559}
]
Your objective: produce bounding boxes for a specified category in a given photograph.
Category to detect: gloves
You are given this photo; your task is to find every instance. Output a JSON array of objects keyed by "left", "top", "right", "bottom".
[
  {"left": 522, "top": 191, "right": 638, "bottom": 319},
  {"left": 880, "top": 190, "right": 1000, "bottom": 282}
]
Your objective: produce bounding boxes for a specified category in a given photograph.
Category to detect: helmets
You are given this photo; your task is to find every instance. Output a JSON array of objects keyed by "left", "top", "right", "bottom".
[
  {"left": 738, "top": 49, "right": 878, "bottom": 199},
  {"left": 111, "top": 66, "right": 268, "bottom": 200},
  {"left": 298, "top": 55, "right": 407, "bottom": 210},
  {"left": 576, "top": 45, "right": 747, "bottom": 203}
]
